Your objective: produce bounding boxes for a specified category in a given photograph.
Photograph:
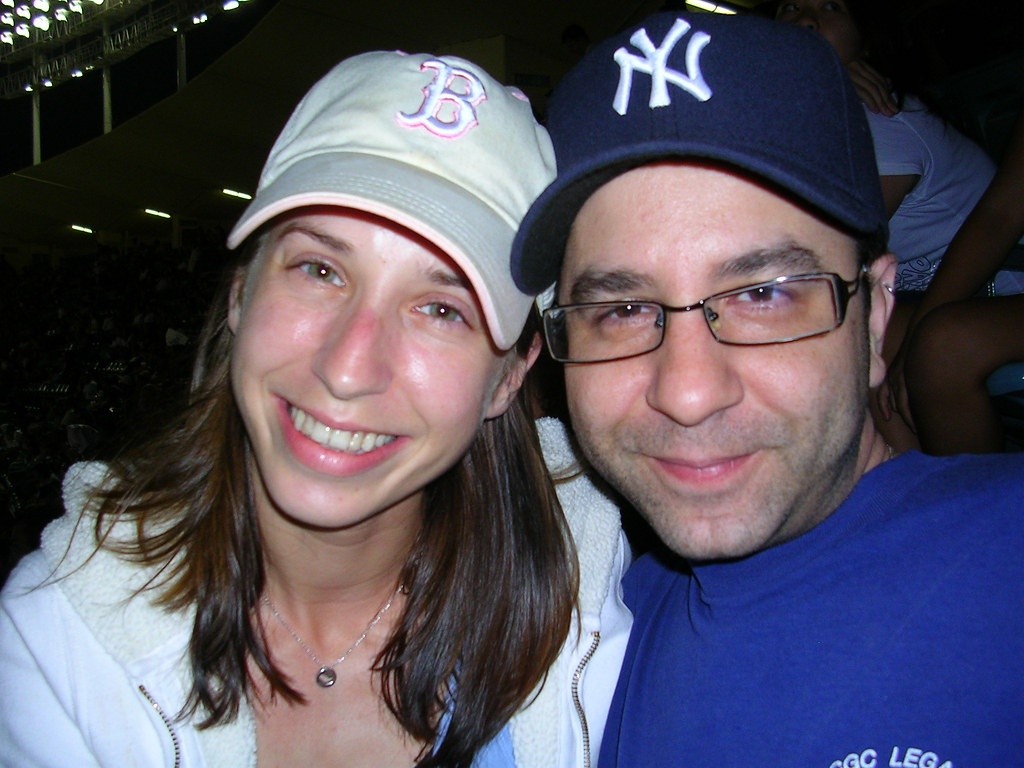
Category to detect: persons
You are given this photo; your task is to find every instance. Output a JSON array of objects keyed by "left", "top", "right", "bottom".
[
  {"left": 0, "top": 50, "right": 634, "bottom": 768},
  {"left": 775, "top": 0, "right": 1024, "bottom": 457},
  {"left": 511, "top": 14, "right": 1024, "bottom": 768}
]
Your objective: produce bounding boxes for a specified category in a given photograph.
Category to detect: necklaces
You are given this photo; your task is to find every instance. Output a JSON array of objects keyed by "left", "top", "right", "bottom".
[{"left": 261, "top": 582, "right": 405, "bottom": 687}]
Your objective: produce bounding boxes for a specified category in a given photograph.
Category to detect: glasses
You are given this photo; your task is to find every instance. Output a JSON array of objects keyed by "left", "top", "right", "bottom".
[{"left": 542, "top": 254, "right": 870, "bottom": 363}]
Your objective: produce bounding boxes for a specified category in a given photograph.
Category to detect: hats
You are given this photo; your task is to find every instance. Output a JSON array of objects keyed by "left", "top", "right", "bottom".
[
  {"left": 510, "top": 13, "right": 888, "bottom": 296},
  {"left": 227, "top": 50, "right": 556, "bottom": 350}
]
